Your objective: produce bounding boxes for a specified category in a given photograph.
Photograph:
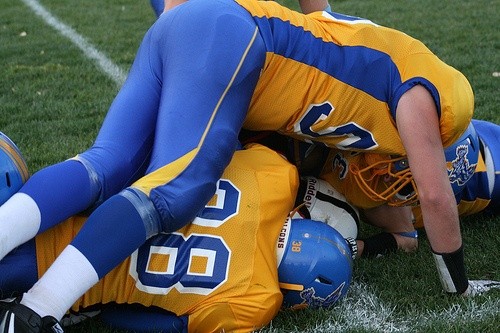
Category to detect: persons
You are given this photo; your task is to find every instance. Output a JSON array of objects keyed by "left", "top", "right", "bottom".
[
  {"left": 32, "top": 142, "right": 353, "bottom": 333},
  {"left": 245, "top": 118, "right": 499, "bottom": 257},
  {"left": 0, "top": 0, "right": 500, "bottom": 333},
  {"left": 150, "top": 0, "right": 334, "bottom": 179}
]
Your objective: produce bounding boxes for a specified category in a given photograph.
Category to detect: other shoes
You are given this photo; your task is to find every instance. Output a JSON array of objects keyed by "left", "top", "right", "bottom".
[{"left": 0, "top": 292, "right": 65, "bottom": 333}]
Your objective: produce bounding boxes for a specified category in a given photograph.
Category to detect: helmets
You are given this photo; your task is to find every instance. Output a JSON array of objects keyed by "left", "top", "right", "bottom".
[
  {"left": 0, "top": 131, "right": 30, "bottom": 208},
  {"left": 349, "top": 153, "right": 419, "bottom": 208},
  {"left": 277, "top": 176, "right": 360, "bottom": 314},
  {"left": 443, "top": 121, "right": 480, "bottom": 196}
]
